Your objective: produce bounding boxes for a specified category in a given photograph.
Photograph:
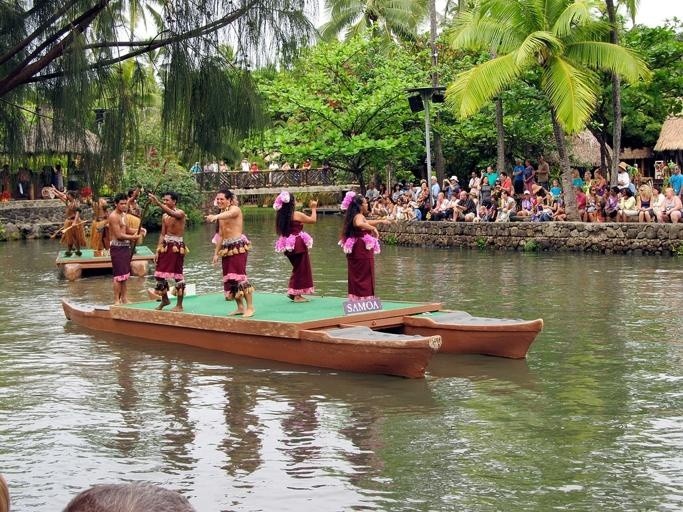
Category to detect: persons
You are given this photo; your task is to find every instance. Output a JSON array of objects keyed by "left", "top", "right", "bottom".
[
  {"left": 190, "top": 158, "right": 330, "bottom": 187},
  {"left": 211, "top": 194, "right": 239, "bottom": 301},
  {"left": 338, "top": 190, "right": 381, "bottom": 301},
  {"left": 109, "top": 193, "right": 146, "bottom": 305},
  {"left": 273, "top": 190, "right": 317, "bottom": 303},
  {"left": 62, "top": 483, "right": 192, "bottom": 512},
  {"left": 146, "top": 191, "right": 190, "bottom": 312},
  {"left": 205, "top": 190, "right": 255, "bottom": 317},
  {"left": 0, "top": 161, "right": 141, "bottom": 256}
]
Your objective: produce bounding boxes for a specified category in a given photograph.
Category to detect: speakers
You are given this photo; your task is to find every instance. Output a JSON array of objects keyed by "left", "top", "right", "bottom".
[
  {"left": 408, "top": 95, "right": 424, "bottom": 113},
  {"left": 431, "top": 95, "right": 445, "bottom": 103}
]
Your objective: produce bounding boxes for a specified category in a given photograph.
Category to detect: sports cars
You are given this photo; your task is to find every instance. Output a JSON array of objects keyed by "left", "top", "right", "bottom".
[{"left": 58, "top": 285, "right": 543, "bottom": 381}]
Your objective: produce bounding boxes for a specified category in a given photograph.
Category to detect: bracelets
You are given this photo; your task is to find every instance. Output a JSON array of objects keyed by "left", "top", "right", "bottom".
[{"left": 157, "top": 202, "right": 161, "bottom": 206}]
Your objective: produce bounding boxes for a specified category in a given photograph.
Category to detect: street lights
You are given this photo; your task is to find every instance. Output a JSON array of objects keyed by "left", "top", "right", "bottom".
[{"left": 405, "top": 87, "right": 443, "bottom": 211}]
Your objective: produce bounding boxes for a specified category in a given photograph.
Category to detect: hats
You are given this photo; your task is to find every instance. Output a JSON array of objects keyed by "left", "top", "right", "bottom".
[
  {"left": 523, "top": 190, "right": 530, "bottom": 195},
  {"left": 618, "top": 162, "right": 629, "bottom": 172},
  {"left": 531, "top": 184, "right": 542, "bottom": 196},
  {"left": 449, "top": 176, "right": 459, "bottom": 183}
]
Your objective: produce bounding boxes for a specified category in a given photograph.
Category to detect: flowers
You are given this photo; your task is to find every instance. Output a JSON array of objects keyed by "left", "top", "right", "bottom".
[
  {"left": 217, "top": 234, "right": 251, "bottom": 257},
  {"left": 270, "top": 189, "right": 291, "bottom": 211},
  {"left": 339, "top": 190, "right": 354, "bottom": 209},
  {"left": 223, "top": 280, "right": 255, "bottom": 303},
  {"left": 156, "top": 241, "right": 190, "bottom": 256},
  {"left": 79, "top": 186, "right": 92, "bottom": 203},
  {"left": 272, "top": 231, "right": 312, "bottom": 253},
  {"left": 337, "top": 233, "right": 381, "bottom": 255},
  {"left": 155, "top": 279, "right": 186, "bottom": 297}
]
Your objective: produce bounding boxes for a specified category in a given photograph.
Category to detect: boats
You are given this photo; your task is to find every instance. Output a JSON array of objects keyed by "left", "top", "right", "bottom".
[{"left": 55, "top": 244, "right": 156, "bottom": 280}]
